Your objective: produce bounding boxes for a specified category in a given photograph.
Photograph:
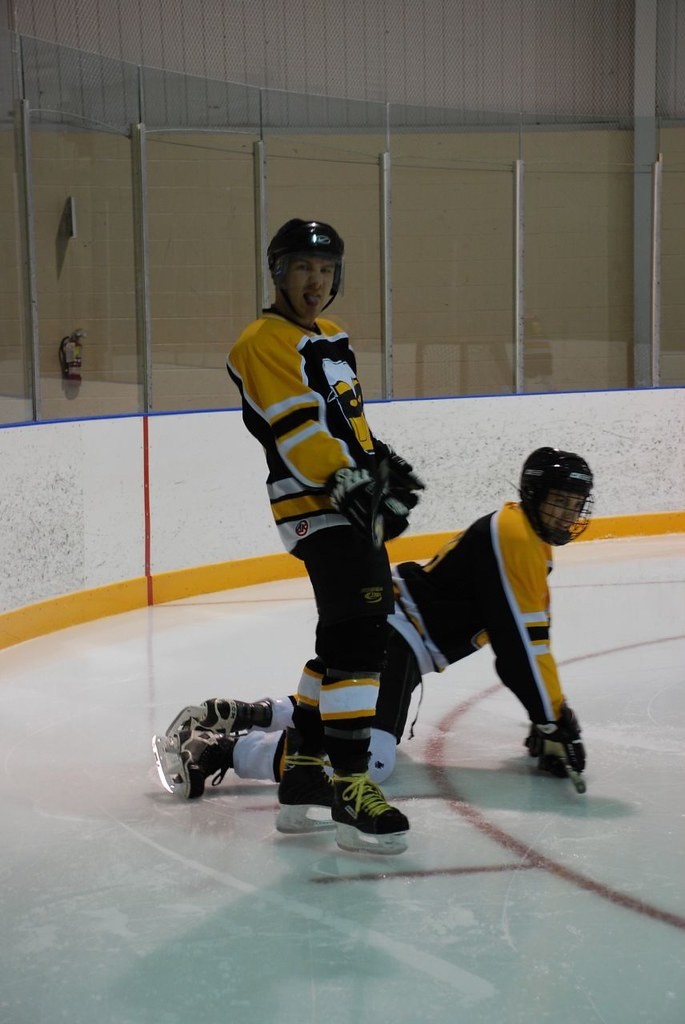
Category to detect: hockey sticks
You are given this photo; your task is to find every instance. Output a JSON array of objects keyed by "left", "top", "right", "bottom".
[{"left": 555, "top": 749, "right": 586, "bottom": 794}]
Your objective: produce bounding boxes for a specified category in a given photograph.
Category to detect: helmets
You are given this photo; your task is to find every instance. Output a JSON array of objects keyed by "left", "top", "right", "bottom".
[
  {"left": 267, "top": 218, "right": 345, "bottom": 296},
  {"left": 521, "top": 446, "right": 594, "bottom": 546}
]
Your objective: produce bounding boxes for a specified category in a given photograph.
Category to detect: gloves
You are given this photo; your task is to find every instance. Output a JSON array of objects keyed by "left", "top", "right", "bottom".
[
  {"left": 523, "top": 706, "right": 586, "bottom": 778},
  {"left": 329, "top": 438, "right": 425, "bottom": 544}
]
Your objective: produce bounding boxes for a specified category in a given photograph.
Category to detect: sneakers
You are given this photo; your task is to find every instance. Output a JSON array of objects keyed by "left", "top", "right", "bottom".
[
  {"left": 276, "top": 728, "right": 337, "bottom": 834},
  {"left": 331, "top": 750, "right": 410, "bottom": 855},
  {"left": 151, "top": 698, "right": 273, "bottom": 799}
]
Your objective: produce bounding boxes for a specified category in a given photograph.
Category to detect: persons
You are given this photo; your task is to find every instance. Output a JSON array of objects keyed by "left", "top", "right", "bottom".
[
  {"left": 224, "top": 216, "right": 429, "bottom": 856},
  {"left": 154, "top": 445, "right": 594, "bottom": 798}
]
